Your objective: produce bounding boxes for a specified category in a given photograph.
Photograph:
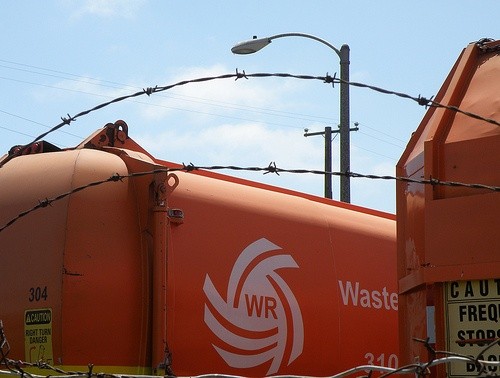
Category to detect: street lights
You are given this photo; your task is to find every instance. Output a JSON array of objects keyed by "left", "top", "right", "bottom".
[{"left": 231, "top": 31, "right": 350, "bottom": 204}]
